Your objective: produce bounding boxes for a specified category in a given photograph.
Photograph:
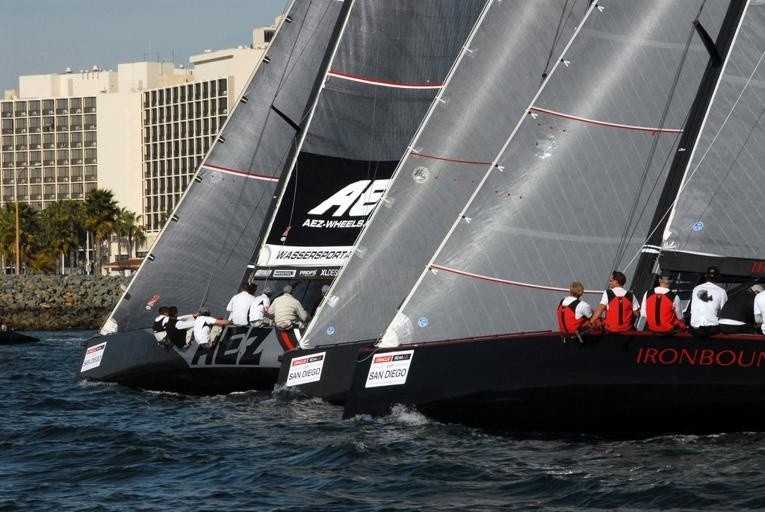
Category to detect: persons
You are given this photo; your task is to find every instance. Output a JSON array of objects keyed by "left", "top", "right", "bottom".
[
  {"left": 248, "top": 286, "right": 275, "bottom": 328},
  {"left": 310, "top": 284, "right": 330, "bottom": 318},
  {"left": 751, "top": 289, "right": 765, "bottom": 336},
  {"left": 266, "top": 284, "right": 308, "bottom": 332},
  {"left": 581, "top": 269, "right": 641, "bottom": 336},
  {"left": 224, "top": 281, "right": 256, "bottom": 328},
  {"left": 150, "top": 306, "right": 175, "bottom": 353},
  {"left": 164, "top": 306, "right": 195, "bottom": 349},
  {"left": 556, "top": 280, "right": 605, "bottom": 336},
  {"left": 639, "top": 270, "right": 689, "bottom": 337},
  {"left": 688, "top": 265, "right": 731, "bottom": 338},
  {"left": 191, "top": 307, "right": 230, "bottom": 349}
]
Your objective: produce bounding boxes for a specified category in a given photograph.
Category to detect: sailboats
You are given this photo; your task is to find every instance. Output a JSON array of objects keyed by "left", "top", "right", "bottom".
[
  {"left": 79, "top": 0, "right": 488, "bottom": 399},
  {"left": 275, "top": 0, "right": 765, "bottom": 440}
]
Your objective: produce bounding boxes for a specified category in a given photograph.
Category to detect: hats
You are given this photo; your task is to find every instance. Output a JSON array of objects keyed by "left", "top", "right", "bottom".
[
  {"left": 284, "top": 286, "right": 293, "bottom": 293},
  {"left": 199, "top": 308, "right": 209, "bottom": 314},
  {"left": 263, "top": 288, "right": 273, "bottom": 294},
  {"left": 322, "top": 285, "right": 329, "bottom": 293},
  {"left": 659, "top": 271, "right": 673, "bottom": 280}
]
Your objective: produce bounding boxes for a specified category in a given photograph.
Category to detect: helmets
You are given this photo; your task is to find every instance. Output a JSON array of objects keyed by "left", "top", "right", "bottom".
[{"left": 706, "top": 266, "right": 720, "bottom": 277}]
